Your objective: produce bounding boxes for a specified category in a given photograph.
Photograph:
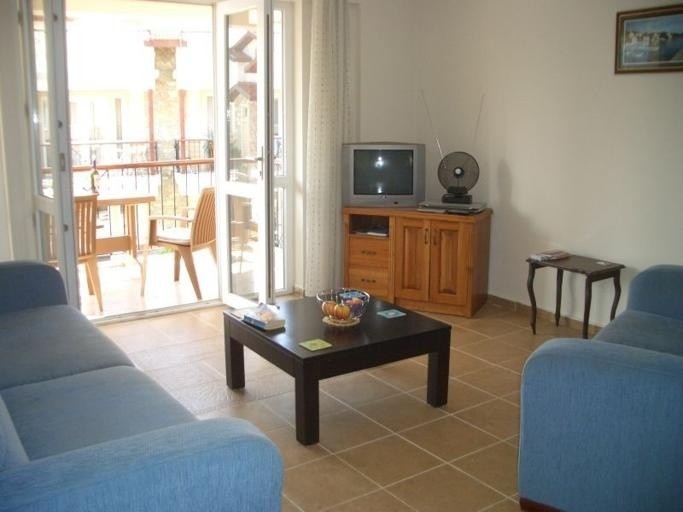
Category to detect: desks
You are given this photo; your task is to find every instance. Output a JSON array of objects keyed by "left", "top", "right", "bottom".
[
  {"left": 526, "top": 255, "right": 624, "bottom": 339},
  {"left": 86, "top": 195, "right": 155, "bottom": 264}
]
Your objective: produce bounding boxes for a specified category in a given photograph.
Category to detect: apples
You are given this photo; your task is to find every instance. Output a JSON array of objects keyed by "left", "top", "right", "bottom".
[
  {"left": 334, "top": 303, "right": 350, "bottom": 319},
  {"left": 322, "top": 301, "right": 336, "bottom": 315},
  {"left": 346, "top": 297, "right": 363, "bottom": 305}
]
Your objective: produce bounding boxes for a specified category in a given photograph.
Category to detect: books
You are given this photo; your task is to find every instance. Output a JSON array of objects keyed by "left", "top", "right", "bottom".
[{"left": 242, "top": 302, "right": 285, "bottom": 331}]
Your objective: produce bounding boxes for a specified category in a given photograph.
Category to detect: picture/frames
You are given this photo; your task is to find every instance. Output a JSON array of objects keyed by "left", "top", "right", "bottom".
[{"left": 615, "top": 4, "right": 683, "bottom": 73}]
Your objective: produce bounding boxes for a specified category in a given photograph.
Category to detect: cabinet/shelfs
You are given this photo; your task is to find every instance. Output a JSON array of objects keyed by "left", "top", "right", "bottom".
[{"left": 342, "top": 208, "right": 490, "bottom": 318}]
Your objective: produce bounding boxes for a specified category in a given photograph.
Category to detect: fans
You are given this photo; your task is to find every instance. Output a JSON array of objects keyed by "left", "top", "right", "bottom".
[{"left": 438, "top": 152, "right": 479, "bottom": 204}]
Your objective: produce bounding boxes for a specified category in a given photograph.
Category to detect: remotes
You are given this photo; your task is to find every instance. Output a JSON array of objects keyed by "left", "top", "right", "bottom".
[{"left": 447, "top": 209, "right": 469, "bottom": 216}]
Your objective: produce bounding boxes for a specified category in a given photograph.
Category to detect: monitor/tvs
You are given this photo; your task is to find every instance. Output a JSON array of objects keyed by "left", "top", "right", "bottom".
[{"left": 341, "top": 141, "right": 427, "bottom": 208}]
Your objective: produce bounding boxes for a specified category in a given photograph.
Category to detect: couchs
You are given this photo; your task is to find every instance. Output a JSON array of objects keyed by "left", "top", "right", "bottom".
[{"left": 0, "top": 261, "right": 283, "bottom": 512}]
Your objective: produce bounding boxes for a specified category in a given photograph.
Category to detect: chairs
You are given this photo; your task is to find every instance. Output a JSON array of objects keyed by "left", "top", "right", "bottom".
[
  {"left": 47, "top": 195, "right": 102, "bottom": 312},
  {"left": 140, "top": 188, "right": 216, "bottom": 301},
  {"left": 517, "top": 265, "right": 683, "bottom": 512}
]
